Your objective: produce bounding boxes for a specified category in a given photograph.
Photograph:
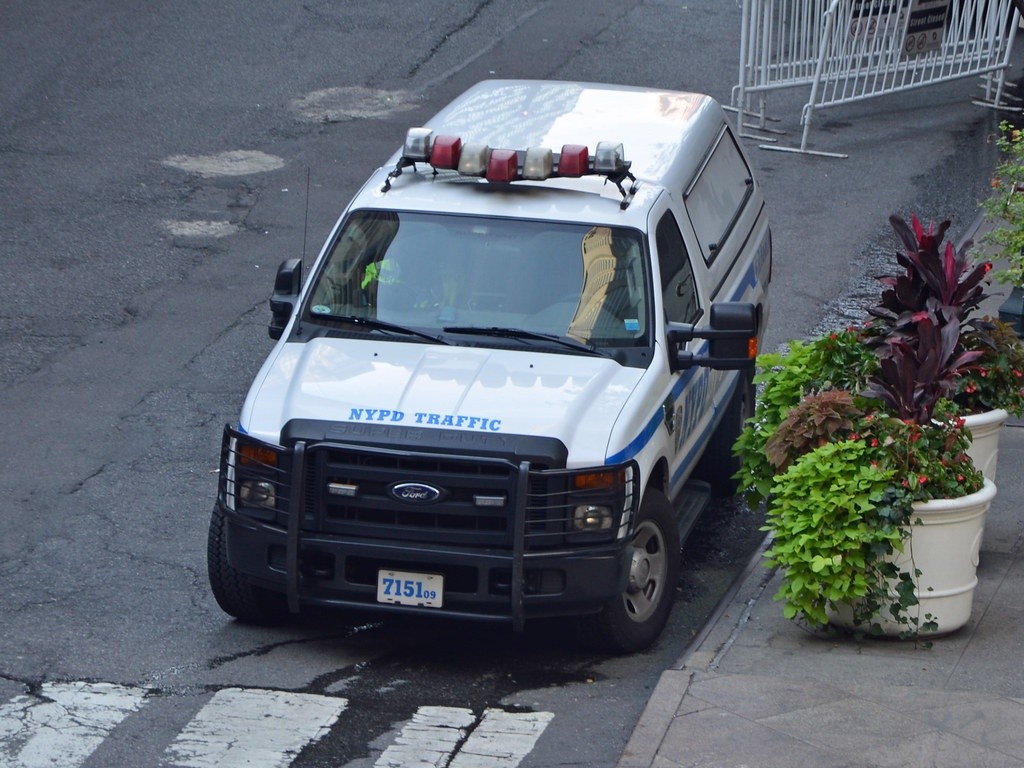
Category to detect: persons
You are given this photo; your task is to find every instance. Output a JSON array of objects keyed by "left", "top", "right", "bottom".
[{"left": 361, "top": 233, "right": 455, "bottom": 313}]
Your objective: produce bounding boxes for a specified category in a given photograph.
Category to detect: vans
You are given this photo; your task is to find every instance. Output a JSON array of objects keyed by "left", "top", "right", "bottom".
[{"left": 206, "top": 77, "right": 773, "bottom": 651}]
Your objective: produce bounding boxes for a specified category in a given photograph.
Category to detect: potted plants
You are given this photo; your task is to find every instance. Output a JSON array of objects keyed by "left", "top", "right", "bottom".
[{"left": 732, "top": 210, "right": 1024, "bottom": 650}]
